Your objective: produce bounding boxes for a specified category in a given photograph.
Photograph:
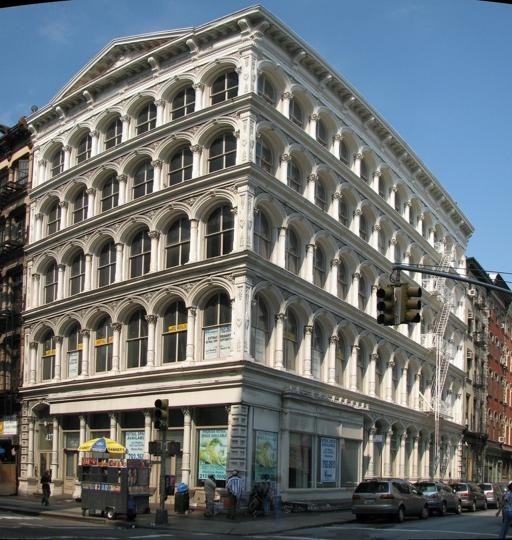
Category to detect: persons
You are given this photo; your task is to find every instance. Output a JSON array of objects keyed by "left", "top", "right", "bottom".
[
  {"left": 204, "top": 473, "right": 216, "bottom": 519},
  {"left": 495, "top": 483, "right": 512, "bottom": 538},
  {"left": 41, "top": 470, "right": 52, "bottom": 506},
  {"left": 261, "top": 474, "right": 272, "bottom": 515},
  {"left": 226, "top": 471, "right": 242, "bottom": 519}
]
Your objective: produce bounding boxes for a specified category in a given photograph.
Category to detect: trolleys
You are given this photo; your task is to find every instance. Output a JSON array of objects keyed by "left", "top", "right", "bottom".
[{"left": 77, "top": 457, "right": 162, "bottom": 519}]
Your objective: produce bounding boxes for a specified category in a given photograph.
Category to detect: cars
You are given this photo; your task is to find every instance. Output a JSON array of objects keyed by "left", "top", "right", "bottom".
[{"left": 352, "top": 478, "right": 511, "bottom": 523}]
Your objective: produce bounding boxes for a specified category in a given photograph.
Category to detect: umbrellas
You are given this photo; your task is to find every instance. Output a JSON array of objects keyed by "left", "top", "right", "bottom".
[{"left": 78, "top": 436, "right": 128, "bottom": 459}]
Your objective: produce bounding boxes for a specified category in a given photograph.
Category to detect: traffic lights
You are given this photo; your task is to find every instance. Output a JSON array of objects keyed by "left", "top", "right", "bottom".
[
  {"left": 373, "top": 286, "right": 396, "bottom": 324},
  {"left": 149, "top": 442, "right": 160, "bottom": 456},
  {"left": 154, "top": 397, "right": 169, "bottom": 432},
  {"left": 165, "top": 442, "right": 180, "bottom": 456},
  {"left": 399, "top": 284, "right": 422, "bottom": 324}
]
